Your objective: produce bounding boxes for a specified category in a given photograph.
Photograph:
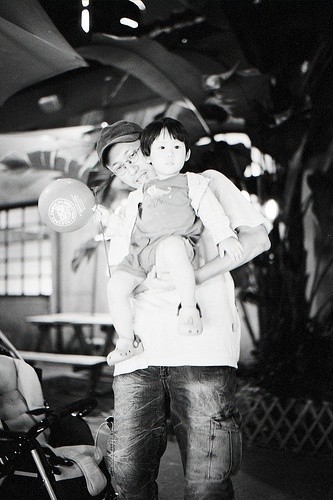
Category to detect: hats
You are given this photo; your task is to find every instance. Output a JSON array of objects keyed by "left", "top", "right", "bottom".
[{"left": 96, "top": 119, "right": 142, "bottom": 169}]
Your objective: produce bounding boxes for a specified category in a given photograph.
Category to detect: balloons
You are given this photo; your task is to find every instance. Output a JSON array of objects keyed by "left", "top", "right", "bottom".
[{"left": 37, "top": 177, "right": 100, "bottom": 235}]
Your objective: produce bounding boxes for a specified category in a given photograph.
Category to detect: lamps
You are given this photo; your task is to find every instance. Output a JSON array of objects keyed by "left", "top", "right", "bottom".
[{"left": 38, "top": 94, "right": 62, "bottom": 115}]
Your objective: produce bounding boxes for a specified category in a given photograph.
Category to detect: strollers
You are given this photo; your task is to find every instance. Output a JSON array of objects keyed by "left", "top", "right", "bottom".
[{"left": 0, "top": 328, "right": 119, "bottom": 500}]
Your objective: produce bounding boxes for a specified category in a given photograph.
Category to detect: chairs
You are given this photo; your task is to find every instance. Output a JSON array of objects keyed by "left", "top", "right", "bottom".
[{"left": 0, "top": 331, "right": 117, "bottom": 500}]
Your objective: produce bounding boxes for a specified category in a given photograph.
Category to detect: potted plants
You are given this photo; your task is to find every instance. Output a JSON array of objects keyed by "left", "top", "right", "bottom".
[{"left": 0, "top": 0, "right": 333, "bottom": 459}]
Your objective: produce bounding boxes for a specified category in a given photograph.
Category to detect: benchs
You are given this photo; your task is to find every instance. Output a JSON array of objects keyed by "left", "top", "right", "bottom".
[{"left": 8, "top": 350, "right": 107, "bottom": 366}]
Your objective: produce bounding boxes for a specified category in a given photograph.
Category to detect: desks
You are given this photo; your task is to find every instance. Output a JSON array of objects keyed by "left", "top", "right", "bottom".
[{"left": 20, "top": 312, "right": 118, "bottom": 401}]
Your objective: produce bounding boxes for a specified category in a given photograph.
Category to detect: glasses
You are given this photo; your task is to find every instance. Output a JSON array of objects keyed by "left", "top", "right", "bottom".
[{"left": 110, "top": 146, "right": 142, "bottom": 178}]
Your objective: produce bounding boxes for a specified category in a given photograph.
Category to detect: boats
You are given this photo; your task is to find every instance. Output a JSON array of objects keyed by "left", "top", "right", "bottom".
[{"left": 13, "top": 313, "right": 119, "bottom": 400}]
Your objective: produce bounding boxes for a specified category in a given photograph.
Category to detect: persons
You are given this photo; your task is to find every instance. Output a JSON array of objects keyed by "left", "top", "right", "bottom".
[
  {"left": 91, "top": 115, "right": 247, "bottom": 368},
  {"left": 100, "top": 120, "right": 272, "bottom": 500}
]
